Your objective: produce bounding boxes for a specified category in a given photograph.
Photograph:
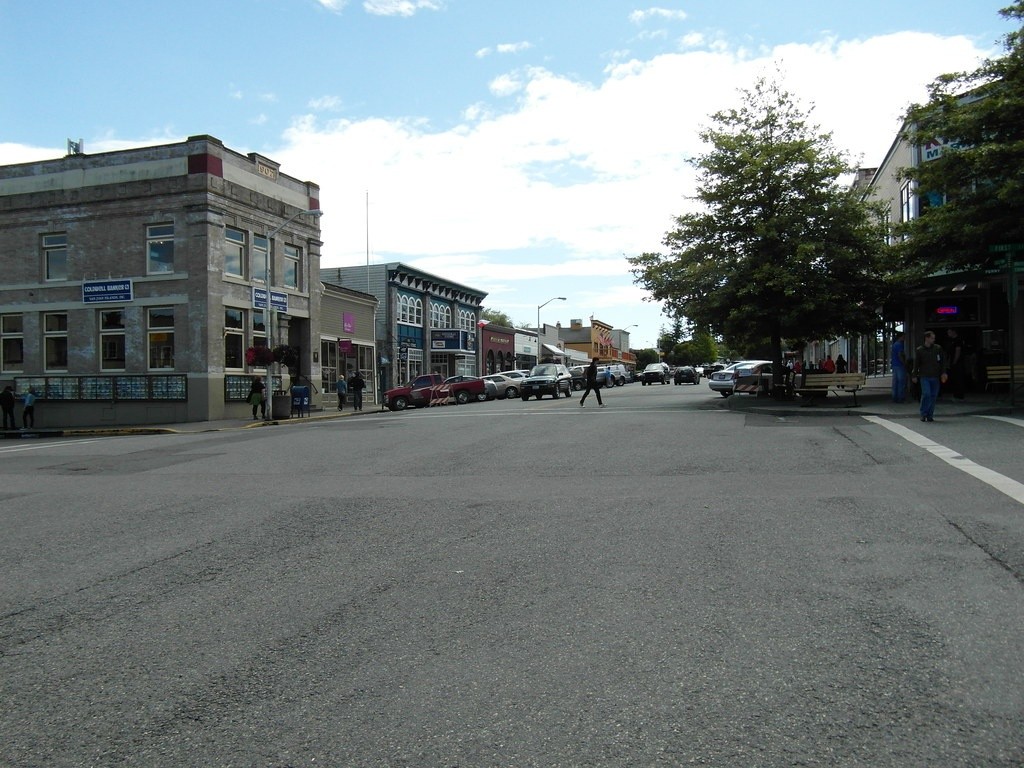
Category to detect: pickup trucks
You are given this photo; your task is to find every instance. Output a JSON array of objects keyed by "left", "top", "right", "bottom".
[{"left": 384, "top": 373, "right": 486, "bottom": 410}]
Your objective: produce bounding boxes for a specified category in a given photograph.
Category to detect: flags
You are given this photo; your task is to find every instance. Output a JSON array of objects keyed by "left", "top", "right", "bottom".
[{"left": 597, "top": 332, "right": 613, "bottom": 346}]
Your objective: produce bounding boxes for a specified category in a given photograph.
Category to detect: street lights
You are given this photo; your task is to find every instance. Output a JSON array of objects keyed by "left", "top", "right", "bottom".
[
  {"left": 619, "top": 325, "right": 639, "bottom": 365},
  {"left": 266, "top": 209, "right": 324, "bottom": 419},
  {"left": 537, "top": 296, "right": 567, "bottom": 365}
]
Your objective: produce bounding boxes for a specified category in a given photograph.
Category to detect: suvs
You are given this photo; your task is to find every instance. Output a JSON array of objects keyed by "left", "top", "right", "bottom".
[
  {"left": 520, "top": 364, "right": 574, "bottom": 401},
  {"left": 606, "top": 365, "right": 631, "bottom": 388},
  {"left": 641, "top": 363, "right": 670, "bottom": 386}
]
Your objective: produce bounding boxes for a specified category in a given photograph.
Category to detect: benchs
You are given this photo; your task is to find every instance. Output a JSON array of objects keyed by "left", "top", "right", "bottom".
[
  {"left": 984, "top": 366, "right": 1024, "bottom": 397},
  {"left": 797, "top": 372, "right": 867, "bottom": 408}
]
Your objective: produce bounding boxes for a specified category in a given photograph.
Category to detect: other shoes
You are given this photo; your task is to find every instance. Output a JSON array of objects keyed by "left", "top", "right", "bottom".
[
  {"left": 896, "top": 400, "right": 910, "bottom": 404},
  {"left": 952, "top": 398, "right": 964, "bottom": 403},
  {"left": 579, "top": 402, "right": 585, "bottom": 408},
  {"left": 921, "top": 415, "right": 934, "bottom": 422},
  {"left": 599, "top": 403, "right": 608, "bottom": 408},
  {"left": 20, "top": 427, "right": 28, "bottom": 430},
  {"left": 337, "top": 407, "right": 341, "bottom": 411}
]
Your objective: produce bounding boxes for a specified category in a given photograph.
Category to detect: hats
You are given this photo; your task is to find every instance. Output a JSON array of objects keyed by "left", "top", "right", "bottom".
[{"left": 339, "top": 374, "right": 344, "bottom": 380}]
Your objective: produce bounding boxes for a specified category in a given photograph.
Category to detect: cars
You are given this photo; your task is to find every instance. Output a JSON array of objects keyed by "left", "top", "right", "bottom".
[
  {"left": 569, "top": 365, "right": 611, "bottom": 390},
  {"left": 480, "top": 375, "right": 523, "bottom": 399},
  {"left": 443, "top": 375, "right": 498, "bottom": 401},
  {"left": 494, "top": 370, "right": 530, "bottom": 378},
  {"left": 708, "top": 360, "right": 802, "bottom": 398},
  {"left": 568, "top": 371, "right": 587, "bottom": 391},
  {"left": 674, "top": 366, "right": 701, "bottom": 385}
]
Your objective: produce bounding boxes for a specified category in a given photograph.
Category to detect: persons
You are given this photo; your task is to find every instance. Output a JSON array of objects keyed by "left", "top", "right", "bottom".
[
  {"left": 23, "top": 387, "right": 36, "bottom": 429},
  {"left": 348, "top": 372, "right": 366, "bottom": 412},
  {"left": 785, "top": 354, "right": 847, "bottom": 390},
  {"left": 0, "top": 386, "right": 17, "bottom": 430},
  {"left": 579, "top": 357, "right": 606, "bottom": 407},
  {"left": 944, "top": 328, "right": 967, "bottom": 402},
  {"left": 250, "top": 376, "right": 267, "bottom": 420},
  {"left": 912, "top": 331, "right": 947, "bottom": 422},
  {"left": 606, "top": 367, "right": 612, "bottom": 388},
  {"left": 335, "top": 375, "right": 347, "bottom": 411},
  {"left": 892, "top": 332, "right": 906, "bottom": 404}
]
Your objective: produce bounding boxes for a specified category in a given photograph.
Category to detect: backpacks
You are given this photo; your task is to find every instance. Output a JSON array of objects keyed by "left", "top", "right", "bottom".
[{"left": 583, "top": 369, "right": 589, "bottom": 378}]
